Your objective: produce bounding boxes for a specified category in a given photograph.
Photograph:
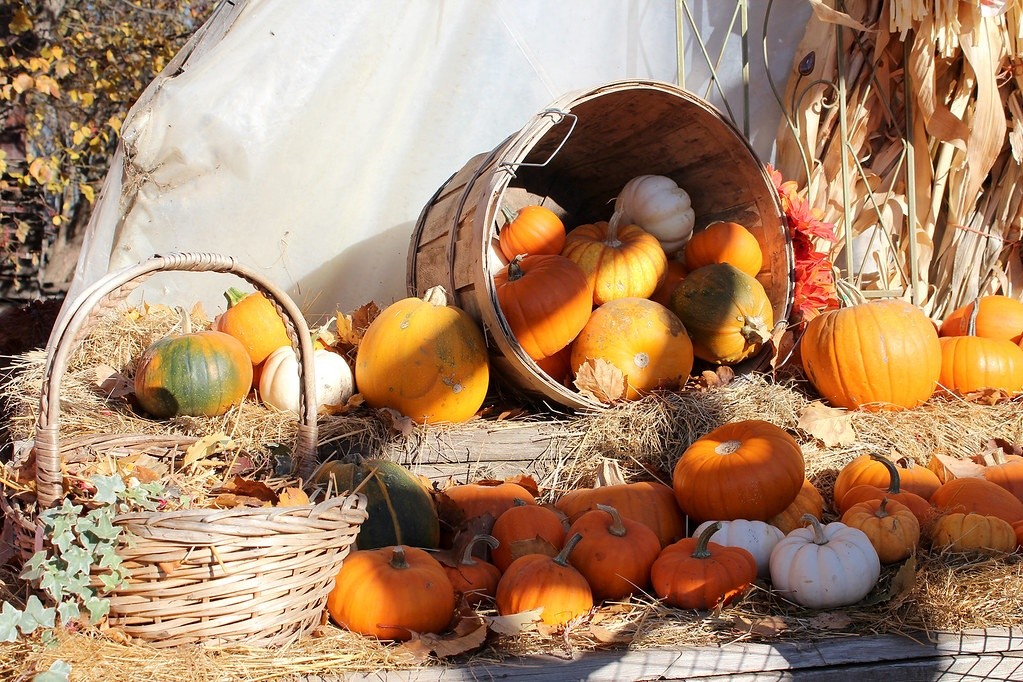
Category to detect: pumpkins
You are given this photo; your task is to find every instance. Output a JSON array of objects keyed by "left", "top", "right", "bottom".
[{"left": 127, "top": 171, "right": 1023, "bottom": 641}]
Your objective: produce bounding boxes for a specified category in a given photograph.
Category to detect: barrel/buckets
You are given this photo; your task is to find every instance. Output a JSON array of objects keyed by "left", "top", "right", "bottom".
[{"left": 405, "top": 77, "right": 795, "bottom": 413}]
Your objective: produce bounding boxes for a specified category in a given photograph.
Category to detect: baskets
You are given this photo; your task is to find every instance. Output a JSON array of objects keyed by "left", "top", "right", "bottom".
[{"left": 0, "top": 253, "right": 367, "bottom": 649}]
